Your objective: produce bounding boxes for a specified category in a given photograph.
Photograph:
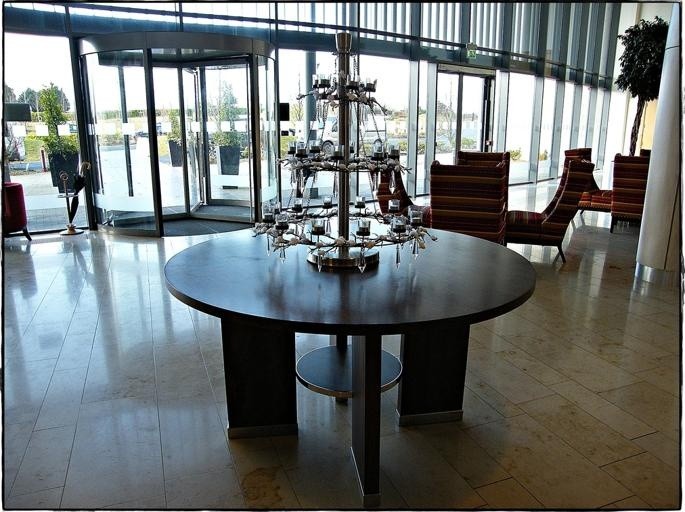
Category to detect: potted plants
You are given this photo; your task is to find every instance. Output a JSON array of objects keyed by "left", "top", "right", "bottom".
[
  {"left": 167, "top": 110, "right": 184, "bottom": 167},
  {"left": 216, "top": 128, "right": 241, "bottom": 175},
  {"left": 38, "top": 135, "right": 78, "bottom": 187}
]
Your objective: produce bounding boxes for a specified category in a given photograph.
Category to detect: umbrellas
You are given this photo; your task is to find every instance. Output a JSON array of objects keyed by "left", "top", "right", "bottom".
[{"left": 70, "top": 162, "right": 91, "bottom": 223}]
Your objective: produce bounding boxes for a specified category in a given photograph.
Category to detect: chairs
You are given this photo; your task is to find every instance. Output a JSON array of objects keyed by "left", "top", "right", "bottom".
[
  {"left": 377, "top": 148, "right": 652, "bottom": 265},
  {"left": 4, "top": 182, "right": 32, "bottom": 241}
]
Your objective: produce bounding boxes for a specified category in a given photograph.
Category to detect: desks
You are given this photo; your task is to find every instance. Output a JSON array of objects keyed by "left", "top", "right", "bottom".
[{"left": 163, "top": 218, "right": 536, "bottom": 508}]
